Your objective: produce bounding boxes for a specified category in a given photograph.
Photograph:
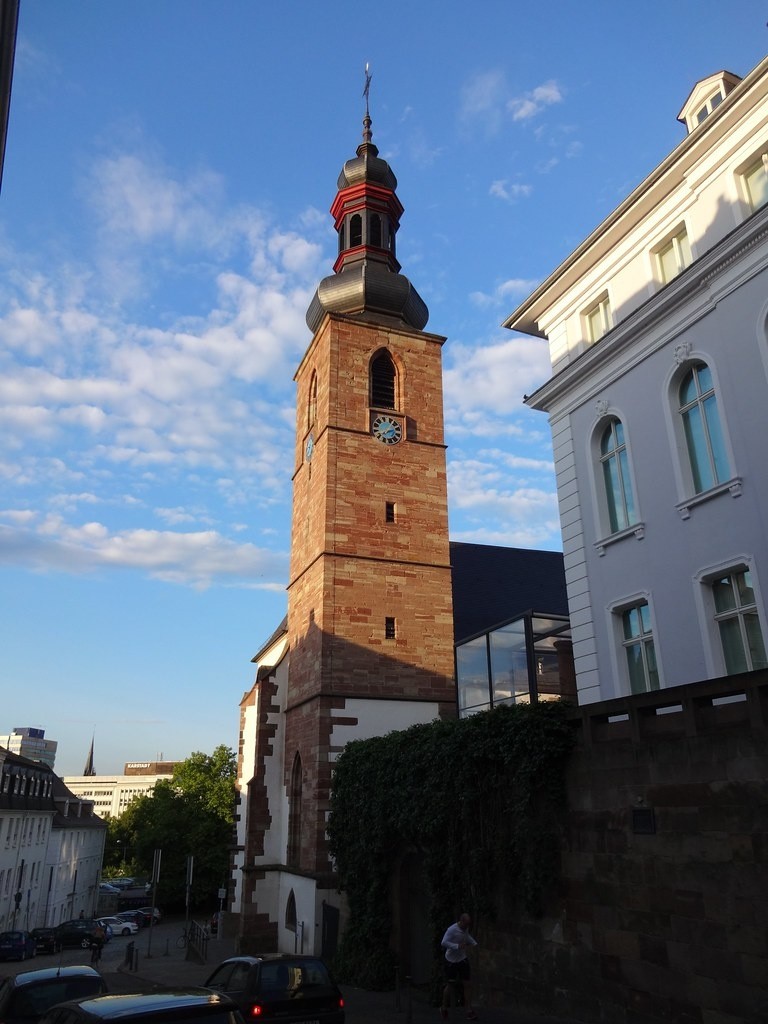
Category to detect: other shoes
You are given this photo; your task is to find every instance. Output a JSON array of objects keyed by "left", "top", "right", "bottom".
[
  {"left": 464, "top": 1011, "right": 478, "bottom": 1020},
  {"left": 438, "top": 1007, "right": 448, "bottom": 1020}
]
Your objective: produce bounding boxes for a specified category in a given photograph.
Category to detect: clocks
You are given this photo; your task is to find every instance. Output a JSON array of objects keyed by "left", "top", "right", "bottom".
[
  {"left": 372, "top": 417, "right": 402, "bottom": 445},
  {"left": 306, "top": 433, "right": 313, "bottom": 462}
]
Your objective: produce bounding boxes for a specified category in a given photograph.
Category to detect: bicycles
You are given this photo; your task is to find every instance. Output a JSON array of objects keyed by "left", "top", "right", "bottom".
[{"left": 176, "top": 927, "right": 201, "bottom": 949}]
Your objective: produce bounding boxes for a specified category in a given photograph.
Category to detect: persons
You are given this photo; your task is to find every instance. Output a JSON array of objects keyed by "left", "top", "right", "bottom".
[
  {"left": 200, "top": 919, "right": 211, "bottom": 940},
  {"left": 439, "top": 912, "right": 479, "bottom": 1021},
  {"left": 122, "top": 940, "right": 135, "bottom": 970},
  {"left": 79, "top": 910, "right": 84, "bottom": 919},
  {"left": 92, "top": 926, "right": 106, "bottom": 961}
]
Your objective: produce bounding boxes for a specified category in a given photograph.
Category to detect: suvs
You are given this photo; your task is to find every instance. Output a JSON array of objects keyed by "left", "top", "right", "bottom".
[{"left": 0, "top": 929, "right": 38, "bottom": 962}]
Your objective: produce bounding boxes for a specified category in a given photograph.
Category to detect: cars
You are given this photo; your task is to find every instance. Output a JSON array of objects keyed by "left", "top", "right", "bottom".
[
  {"left": 197, "top": 952, "right": 347, "bottom": 1024},
  {"left": 36, "top": 985, "right": 248, "bottom": 1024},
  {"left": 0, "top": 962, "right": 114, "bottom": 1024},
  {"left": 31, "top": 906, "right": 162, "bottom": 954}
]
